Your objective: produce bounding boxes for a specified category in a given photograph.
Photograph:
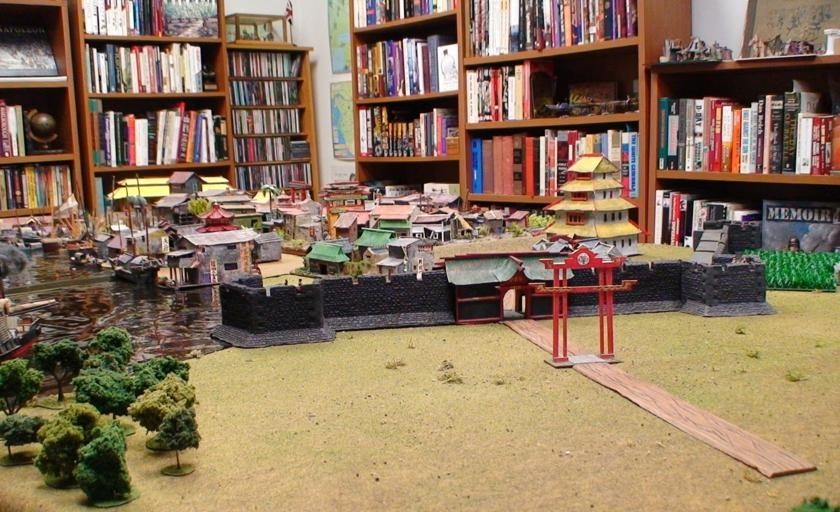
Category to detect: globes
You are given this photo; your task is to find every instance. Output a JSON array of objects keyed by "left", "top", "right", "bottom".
[{"left": 24, "top": 108, "right": 63, "bottom": 154}]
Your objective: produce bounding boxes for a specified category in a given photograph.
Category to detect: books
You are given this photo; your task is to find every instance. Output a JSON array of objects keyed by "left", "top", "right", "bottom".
[
  {"left": 352, "top": 0, "right": 460, "bottom": 158},
  {"left": 222, "top": 48, "right": 313, "bottom": 200},
  {"left": 80, "top": 0, "right": 234, "bottom": 219},
  {"left": 462, "top": 0, "right": 641, "bottom": 243},
  {"left": 654, "top": 96, "right": 839, "bottom": 251},
  {"left": 1, "top": 19, "right": 71, "bottom": 212}
]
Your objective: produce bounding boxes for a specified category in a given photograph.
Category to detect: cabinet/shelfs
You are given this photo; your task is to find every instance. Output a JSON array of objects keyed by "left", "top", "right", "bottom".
[
  {"left": 70, "top": 0, "right": 238, "bottom": 217},
  {"left": 350, "top": 1, "right": 467, "bottom": 247},
  {"left": 461, "top": 1, "right": 694, "bottom": 248},
  {"left": 225, "top": 12, "right": 288, "bottom": 44},
  {"left": 2, "top": 0, "right": 87, "bottom": 216},
  {"left": 648, "top": 52, "right": 839, "bottom": 248},
  {"left": 225, "top": 42, "right": 321, "bottom": 216}
]
